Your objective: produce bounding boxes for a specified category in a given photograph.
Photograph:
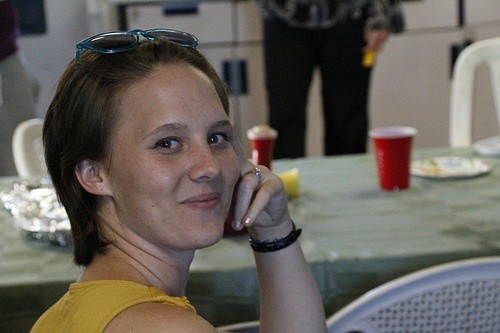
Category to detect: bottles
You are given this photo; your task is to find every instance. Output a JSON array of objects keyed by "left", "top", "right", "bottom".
[{"left": 362, "top": 32, "right": 376, "bottom": 68}]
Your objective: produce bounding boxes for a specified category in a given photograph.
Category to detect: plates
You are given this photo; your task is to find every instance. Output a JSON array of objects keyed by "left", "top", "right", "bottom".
[
  {"left": 409, "top": 156, "right": 493, "bottom": 177},
  {"left": 472, "top": 134, "right": 500, "bottom": 154}
]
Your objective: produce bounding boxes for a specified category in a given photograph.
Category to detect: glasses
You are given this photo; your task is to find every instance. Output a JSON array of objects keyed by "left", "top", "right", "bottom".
[{"left": 75, "top": 28, "right": 199, "bottom": 63}]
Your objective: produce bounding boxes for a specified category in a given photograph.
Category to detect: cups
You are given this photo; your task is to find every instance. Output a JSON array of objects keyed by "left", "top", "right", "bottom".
[
  {"left": 246, "top": 125, "right": 278, "bottom": 170},
  {"left": 277, "top": 168, "right": 300, "bottom": 197},
  {"left": 368, "top": 125, "right": 419, "bottom": 191}
]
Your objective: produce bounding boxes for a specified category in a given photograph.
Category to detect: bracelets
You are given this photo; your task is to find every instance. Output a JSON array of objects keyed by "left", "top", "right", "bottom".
[{"left": 248, "top": 220, "right": 302, "bottom": 252}]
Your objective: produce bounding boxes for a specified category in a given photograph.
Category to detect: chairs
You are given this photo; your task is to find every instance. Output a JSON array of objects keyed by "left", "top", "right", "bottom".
[
  {"left": 450, "top": 36, "right": 500, "bottom": 146},
  {"left": 11, "top": 118, "right": 48, "bottom": 176},
  {"left": 326, "top": 256, "right": 500, "bottom": 333}
]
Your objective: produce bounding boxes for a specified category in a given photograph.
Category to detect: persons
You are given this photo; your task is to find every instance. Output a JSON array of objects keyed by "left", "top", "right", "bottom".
[
  {"left": 31, "top": 29, "right": 327, "bottom": 333},
  {"left": 255, "top": 1, "right": 405, "bottom": 159}
]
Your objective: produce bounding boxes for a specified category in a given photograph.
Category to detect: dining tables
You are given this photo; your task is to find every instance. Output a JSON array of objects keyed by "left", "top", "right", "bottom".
[{"left": 0, "top": 146, "right": 500, "bottom": 315}]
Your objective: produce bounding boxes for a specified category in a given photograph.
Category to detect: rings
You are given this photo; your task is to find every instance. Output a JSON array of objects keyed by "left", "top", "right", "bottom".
[{"left": 245, "top": 167, "right": 262, "bottom": 184}]
